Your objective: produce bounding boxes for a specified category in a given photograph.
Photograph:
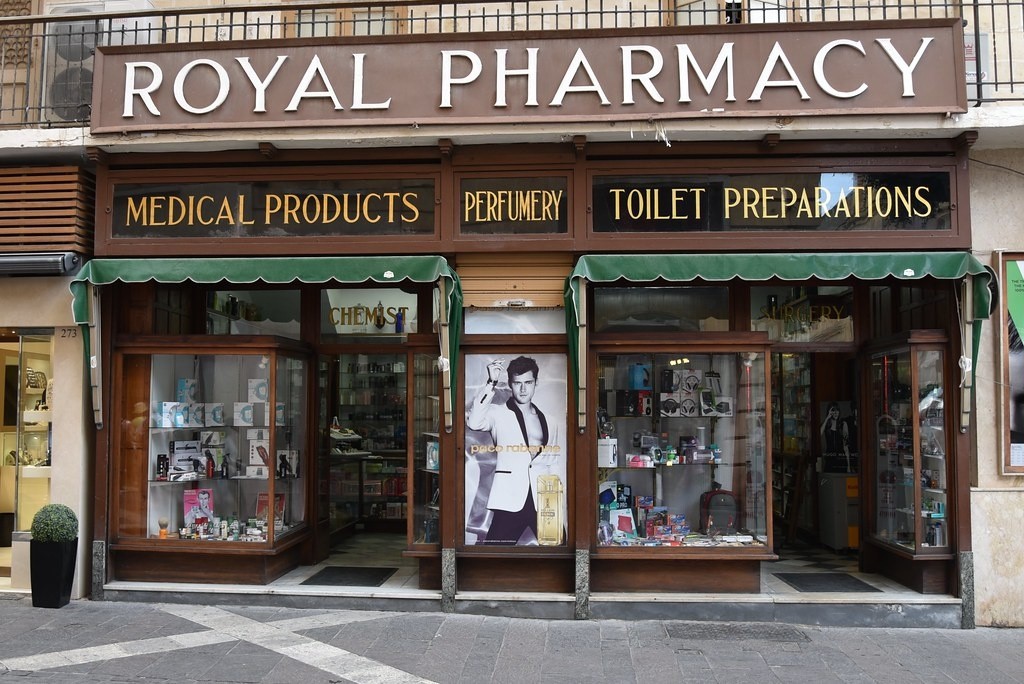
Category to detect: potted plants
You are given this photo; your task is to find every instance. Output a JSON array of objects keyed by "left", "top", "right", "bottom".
[{"left": 29, "top": 505, "right": 79, "bottom": 609}]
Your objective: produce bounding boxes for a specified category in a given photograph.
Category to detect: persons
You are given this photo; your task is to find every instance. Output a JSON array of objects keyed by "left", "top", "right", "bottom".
[
  {"left": 186, "top": 491, "right": 212, "bottom": 531},
  {"left": 466, "top": 355, "right": 569, "bottom": 549},
  {"left": 819, "top": 401, "right": 859, "bottom": 470}
]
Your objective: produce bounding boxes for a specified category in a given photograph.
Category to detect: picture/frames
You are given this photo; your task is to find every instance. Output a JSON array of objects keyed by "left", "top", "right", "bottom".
[
  {"left": 993, "top": 249, "right": 1024, "bottom": 478},
  {"left": 459, "top": 348, "right": 578, "bottom": 554}
]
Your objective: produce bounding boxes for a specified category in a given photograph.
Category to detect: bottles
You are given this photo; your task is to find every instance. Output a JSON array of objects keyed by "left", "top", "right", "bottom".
[{"left": 696, "top": 427, "right": 706, "bottom": 451}]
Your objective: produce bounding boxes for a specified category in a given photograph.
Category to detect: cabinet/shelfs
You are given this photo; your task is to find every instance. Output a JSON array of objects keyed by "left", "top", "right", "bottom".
[
  {"left": 772, "top": 352, "right": 856, "bottom": 542},
  {"left": 319, "top": 354, "right": 408, "bottom": 546}
]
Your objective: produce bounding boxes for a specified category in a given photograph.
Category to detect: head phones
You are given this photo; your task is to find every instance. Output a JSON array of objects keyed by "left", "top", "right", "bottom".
[
  {"left": 684, "top": 375, "right": 700, "bottom": 391},
  {"left": 682, "top": 399, "right": 696, "bottom": 414},
  {"left": 663, "top": 398, "right": 677, "bottom": 413}
]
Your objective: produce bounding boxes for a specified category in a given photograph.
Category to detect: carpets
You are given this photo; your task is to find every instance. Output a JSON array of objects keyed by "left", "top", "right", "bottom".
[
  {"left": 301, "top": 566, "right": 399, "bottom": 588},
  {"left": 771, "top": 572, "right": 884, "bottom": 593}
]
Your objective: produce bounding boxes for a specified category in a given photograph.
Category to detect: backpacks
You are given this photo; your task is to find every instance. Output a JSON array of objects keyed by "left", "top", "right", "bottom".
[{"left": 700, "top": 489, "right": 739, "bottom": 535}]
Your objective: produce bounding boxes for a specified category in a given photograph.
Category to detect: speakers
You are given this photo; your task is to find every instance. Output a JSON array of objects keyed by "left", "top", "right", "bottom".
[
  {"left": 642, "top": 397, "right": 652, "bottom": 416},
  {"left": 661, "top": 370, "right": 674, "bottom": 392}
]
